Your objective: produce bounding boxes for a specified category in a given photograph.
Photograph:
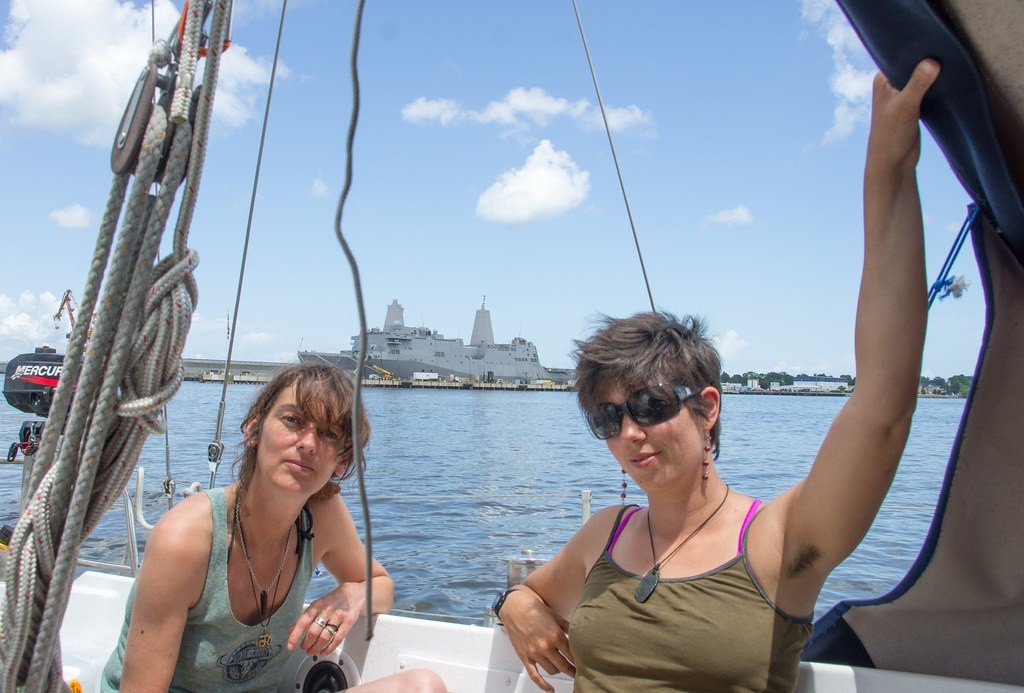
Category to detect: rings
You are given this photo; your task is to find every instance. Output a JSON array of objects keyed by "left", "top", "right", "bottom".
[
  {"left": 326, "top": 624, "right": 338, "bottom": 634},
  {"left": 314, "top": 618, "right": 325, "bottom": 627}
]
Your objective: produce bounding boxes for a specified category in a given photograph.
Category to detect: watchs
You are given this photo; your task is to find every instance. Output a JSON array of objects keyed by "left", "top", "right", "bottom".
[{"left": 492, "top": 588, "right": 517, "bottom": 624}]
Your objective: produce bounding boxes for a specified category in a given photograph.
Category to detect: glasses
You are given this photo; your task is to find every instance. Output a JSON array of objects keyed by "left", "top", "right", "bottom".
[{"left": 584, "top": 383, "right": 707, "bottom": 440}]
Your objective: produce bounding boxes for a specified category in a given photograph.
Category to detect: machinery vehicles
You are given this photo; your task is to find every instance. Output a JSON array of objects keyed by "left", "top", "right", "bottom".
[{"left": 368, "top": 364, "right": 394, "bottom": 381}]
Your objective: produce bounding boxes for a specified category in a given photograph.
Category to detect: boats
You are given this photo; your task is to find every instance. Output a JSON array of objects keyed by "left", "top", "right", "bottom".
[{"left": 296, "top": 299, "right": 568, "bottom": 379}]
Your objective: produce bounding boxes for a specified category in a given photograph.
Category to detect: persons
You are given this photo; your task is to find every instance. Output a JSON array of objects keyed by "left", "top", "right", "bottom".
[
  {"left": 103, "top": 364, "right": 448, "bottom": 693},
  {"left": 496, "top": 55, "right": 940, "bottom": 693}
]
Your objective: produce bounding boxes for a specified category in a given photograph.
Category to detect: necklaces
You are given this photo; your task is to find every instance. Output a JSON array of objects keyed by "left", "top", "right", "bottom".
[
  {"left": 635, "top": 486, "right": 730, "bottom": 603},
  {"left": 234, "top": 491, "right": 293, "bottom": 647}
]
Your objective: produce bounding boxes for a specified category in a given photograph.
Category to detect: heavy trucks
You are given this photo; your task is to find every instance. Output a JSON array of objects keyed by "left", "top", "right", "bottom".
[{"left": 413, "top": 371, "right": 443, "bottom": 381}]
